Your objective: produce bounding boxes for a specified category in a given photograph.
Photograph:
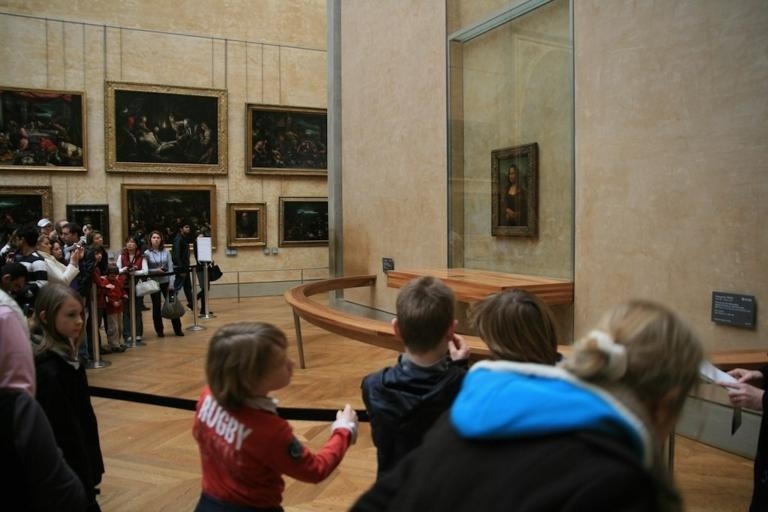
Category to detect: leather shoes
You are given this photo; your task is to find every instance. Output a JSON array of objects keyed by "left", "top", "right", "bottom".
[
  {"left": 158, "top": 331, "right": 163, "bottom": 337},
  {"left": 175, "top": 330, "right": 184, "bottom": 336}
]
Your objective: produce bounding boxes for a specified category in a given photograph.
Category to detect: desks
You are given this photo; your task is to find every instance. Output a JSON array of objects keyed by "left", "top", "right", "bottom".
[{"left": 387, "top": 268, "right": 573, "bottom": 316}]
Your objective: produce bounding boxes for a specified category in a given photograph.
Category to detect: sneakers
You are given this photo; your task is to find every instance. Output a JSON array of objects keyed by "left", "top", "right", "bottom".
[{"left": 100, "top": 336, "right": 142, "bottom": 355}]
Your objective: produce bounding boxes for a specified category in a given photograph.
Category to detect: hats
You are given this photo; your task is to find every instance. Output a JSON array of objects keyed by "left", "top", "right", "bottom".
[{"left": 37, "top": 218, "right": 52, "bottom": 228}]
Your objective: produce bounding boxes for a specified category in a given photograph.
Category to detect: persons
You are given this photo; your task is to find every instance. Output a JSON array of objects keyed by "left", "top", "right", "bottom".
[
  {"left": 0, "top": 119, "right": 82, "bottom": 166},
  {"left": 360, "top": 276, "right": 470, "bottom": 482},
  {"left": 118, "top": 107, "right": 217, "bottom": 164},
  {"left": 251, "top": 128, "right": 327, "bottom": 169},
  {"left": 193, "top": 323, "right": 359, "bottom": 512},
  {"left": 130, "top": 197, "right": 208, "bottom": 242},
  {"left": 716, "top": 365, "right": 767, "bottom": 511},
  {"left": 353, "top": 300, "right": 703, "bottom": 511},
  {"left": 286, "top": 212, "right": 328, "bottom": 241},
  {"left": 503, "top": 164, "right": 527, "bottom": 226},
  {"left": 236, "top": 212, "right": 257, "bottom": 237},
  {"left": 467, "top": 289, "right": 562, "bottom": 369},
  {"left": 116, "top": 219, "right": 211, "bottom": 343},
  {"left": 1, "top": 218, "right": 127, "bottom": 512}
]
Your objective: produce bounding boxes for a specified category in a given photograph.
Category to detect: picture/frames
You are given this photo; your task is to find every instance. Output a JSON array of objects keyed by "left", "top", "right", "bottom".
[
  {"left": 279, "top": 197, "right": 330, "bottom": 247},
  {"left": 244, "top": 103, "right": 328, "bottom": 176},
  {"left": 490, "top": 143, "right": 538, "bottom": 238},
  {"left": 227, "top": 202, "right": 267, "bottom": 247},
  {"left": 103, "top": 81, "right": 228, "bottom": 176},
  {"left": 0, "top": 185, "right": 52, "bottom": 247},
  {"left": 65, "top": 204, "right": 110, "bottom": 250},
  {"left": 1, "top": 85, "right": 89, "bottom": 172},
  {"left": 121, "top": 183, "right": 218, "bottom": 250}
]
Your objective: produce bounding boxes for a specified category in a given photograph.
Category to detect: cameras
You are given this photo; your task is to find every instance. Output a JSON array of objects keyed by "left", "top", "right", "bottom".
[
  {"left": 73, "top": 243, "right": 82, "bottom": 249},
  {"left": 128, "top": 263, "right": 133, "bottom": 271}
]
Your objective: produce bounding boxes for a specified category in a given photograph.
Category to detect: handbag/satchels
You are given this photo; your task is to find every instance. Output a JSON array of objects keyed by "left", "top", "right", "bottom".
[
  {"left": 160, "top": 289, "right": 186, "bottom": 319},
  {"left": 136, "top": 278, "right": 160, "bottom": 297},
  {"left": 207, "top": 260, "right": 222, "bottom": 281}
]
